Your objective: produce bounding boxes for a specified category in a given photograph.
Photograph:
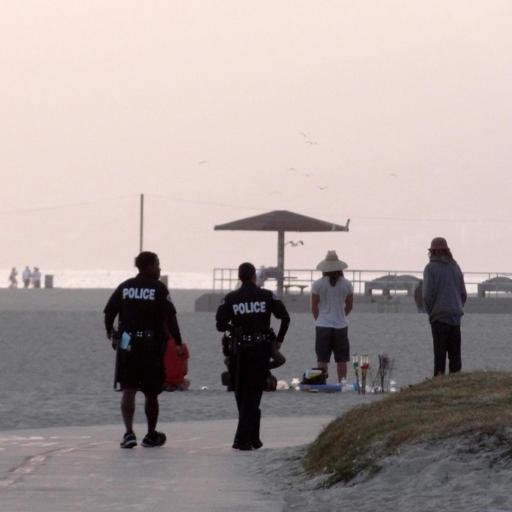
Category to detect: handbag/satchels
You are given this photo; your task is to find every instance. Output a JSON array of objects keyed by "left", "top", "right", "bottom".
[{"left": 301, "top": 367, "right": 328, "bottom": 385}]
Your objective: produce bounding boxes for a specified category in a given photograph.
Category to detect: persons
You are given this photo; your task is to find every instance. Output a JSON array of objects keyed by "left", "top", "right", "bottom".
[
  {"left": 216, "top": 262, "right": 290, "bottom": 451},
  {"left": 423, "top": 237, "right": 467, "bottom": 377},
  {"left": 312, "top": 250, "right": 353, "bottom": 383},
  {"left": 415, "top": 280, "right": 426, "bottom": 313},
  {"left": 103, "top": 251, "right": 182, "bottom": 448},
  {"left": 8, "top": 266, "right": 41, "bottom": 289}
]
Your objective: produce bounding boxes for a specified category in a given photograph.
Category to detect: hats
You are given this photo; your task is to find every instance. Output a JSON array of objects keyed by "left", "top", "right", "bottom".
[
  {"left": 317, "top": 251, "right": 348, "bottom": 272},
  {"left": 428, "top": 238, "right": 448, "bottom": 249}
]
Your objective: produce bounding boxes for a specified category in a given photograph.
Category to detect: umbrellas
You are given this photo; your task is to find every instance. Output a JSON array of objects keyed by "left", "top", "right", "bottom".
[{"left": 215, "top": 210, "right": 350, "bottom": 300}]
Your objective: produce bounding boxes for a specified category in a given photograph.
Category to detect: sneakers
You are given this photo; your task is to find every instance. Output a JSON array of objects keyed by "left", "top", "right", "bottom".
[
  {"left": 120, "top": 432, "right": 137, "bottom": 448},
  {"left": 141, "top": 431, "right": 166, "bottom": 448}
]
